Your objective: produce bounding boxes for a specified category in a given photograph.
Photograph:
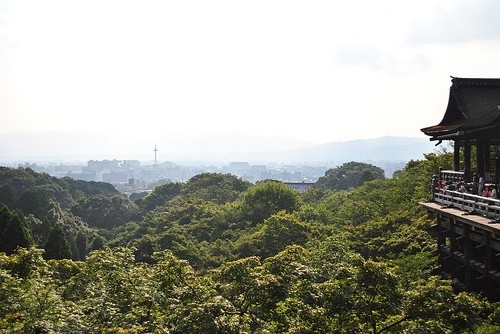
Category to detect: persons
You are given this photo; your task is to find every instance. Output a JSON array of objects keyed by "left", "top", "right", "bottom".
[{"left": 437, "top": 178, "right": 500, "bottom": 201}]
